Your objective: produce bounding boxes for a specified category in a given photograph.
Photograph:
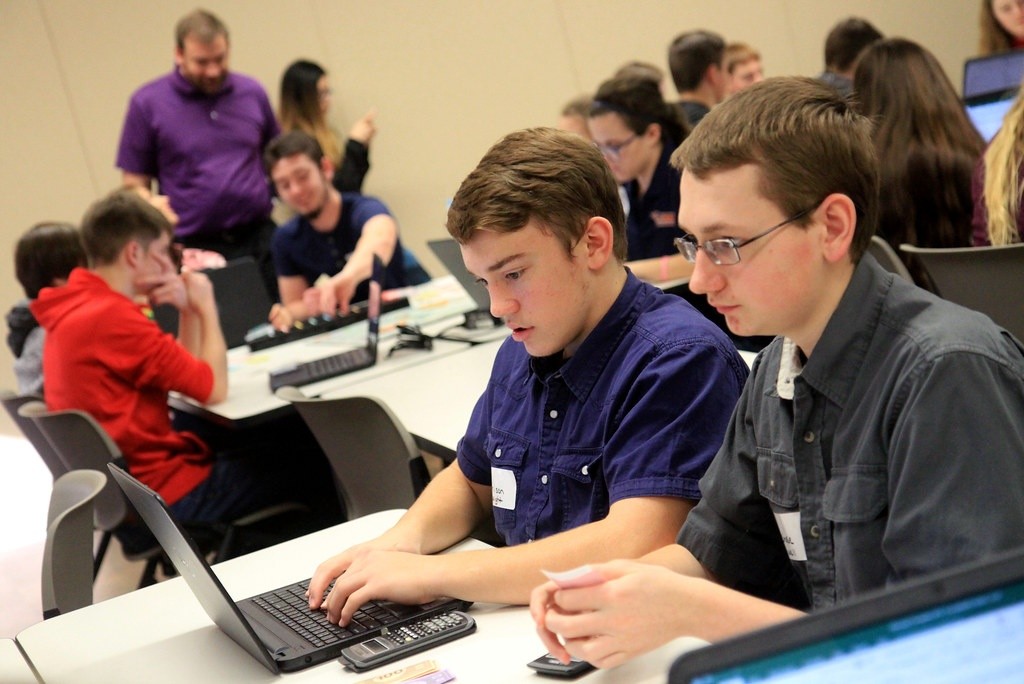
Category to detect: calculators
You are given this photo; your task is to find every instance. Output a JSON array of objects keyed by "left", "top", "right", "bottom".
[
  {"left": 340, "top": 609, "right": 477, "bottom": 670},
  {"left": 525, "top": 652, "right": 595, "bottom": 677}
]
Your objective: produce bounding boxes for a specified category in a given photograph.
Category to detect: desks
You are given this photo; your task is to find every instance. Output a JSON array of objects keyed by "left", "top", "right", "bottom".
[{"left": 0, "top": 276, "right": 713, "bottom": 684}]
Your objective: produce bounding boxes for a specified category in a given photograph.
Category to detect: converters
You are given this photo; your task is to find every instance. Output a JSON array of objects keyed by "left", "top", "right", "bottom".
[{"left": 463, "top": 308, "right": 502, "bottom": 329}]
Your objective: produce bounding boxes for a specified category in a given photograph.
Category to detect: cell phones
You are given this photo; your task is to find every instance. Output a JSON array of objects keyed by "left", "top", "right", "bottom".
[{"left": 527, "top": 652, "right": 591, "bottom": 676}]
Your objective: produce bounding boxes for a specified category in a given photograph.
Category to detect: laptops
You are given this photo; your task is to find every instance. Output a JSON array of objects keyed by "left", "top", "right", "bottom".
[
  {"left": 269, "top": 279, "right": 383, "bottom": 395},
  {"left": 669, "top": 544, "right": 1024, "bottom": 684},
  {"left": 429, "top": 238, "right": 505, "bottom": 328},
  {"left": 963, "top": 48, "right": 1024, "bottom": 145},
  {"left": 107, "top": 462, "right": 476, "bottom": 676}
]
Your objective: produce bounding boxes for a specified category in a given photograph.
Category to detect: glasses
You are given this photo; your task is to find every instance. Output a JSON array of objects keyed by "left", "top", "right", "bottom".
[
  {"left": 592, "top": 133, "right": 640, "bottom": 158},
  {"left": 675, "top": 200, "right": 826, "bottom": 266}
]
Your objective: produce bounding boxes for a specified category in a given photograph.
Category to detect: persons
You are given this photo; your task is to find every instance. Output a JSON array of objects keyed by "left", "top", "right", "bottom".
[
  {"left": 5, "top": 191, "right": 349, "bottom": 534},
  {"left": 853, "top": 38, "right": 985, "bottom": 249},
  {"left": 118, "top": 10, "right": 434, "bottom": 334},
  {"left": 974, "top": 84, "right": 1024, "bottom": 246},
  {"left": 979, "top": 0, "right": 1024, "bottom": 56},
  {"left": 530, "top": 76, "right": 1023, "bottom": 669},
  {"left": 306, "top": 124, "right": 750, "bottom": 631},
  {"left": 823, "top": 17, "right": 883, "bottom": 86},
  {"left": 561, "top": 30, "right": 766, "bottom": 262}
]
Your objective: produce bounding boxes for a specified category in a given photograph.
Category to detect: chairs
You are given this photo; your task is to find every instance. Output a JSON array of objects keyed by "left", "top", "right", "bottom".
[
  {"left": 17, "top": 400, "right": 234, "bottom": 589},
  {"left": 865, "top": 235, "right": 1023, "bottom": 350},
  {"left": 0, "top": 390, "right": 112, "bottom": 586},
  {"left": 41, "top": 469, "right": 107, "bottom": 620},
  {"left": 274, "top": 387, "right": 431, "bottom": 520}
]
